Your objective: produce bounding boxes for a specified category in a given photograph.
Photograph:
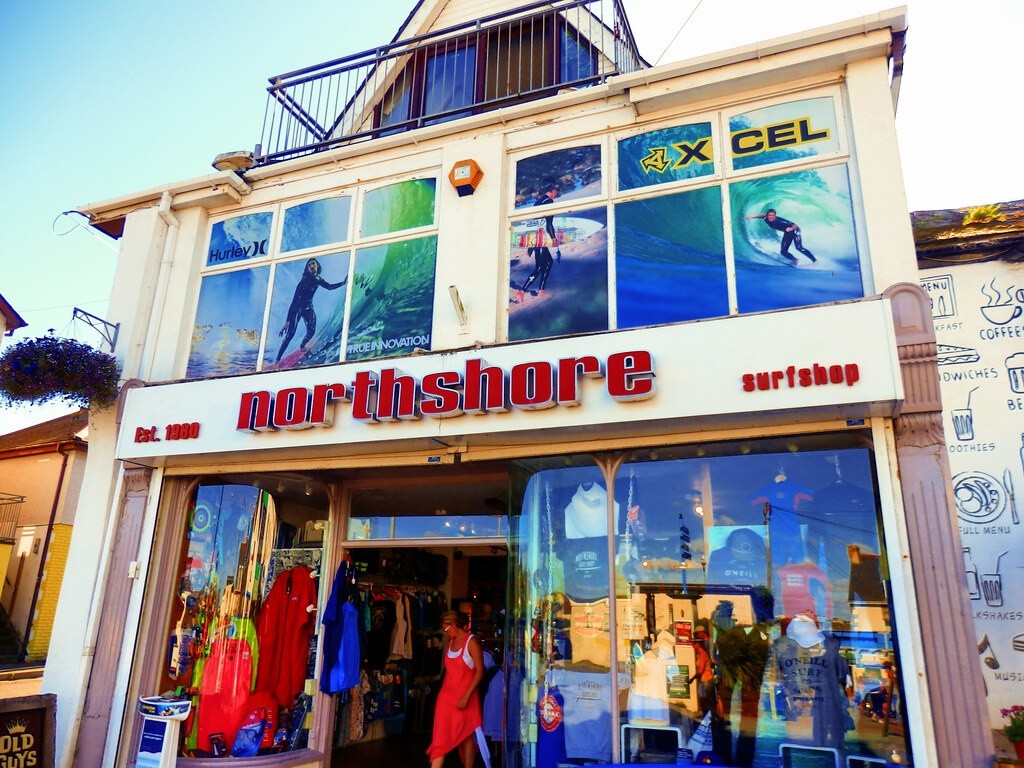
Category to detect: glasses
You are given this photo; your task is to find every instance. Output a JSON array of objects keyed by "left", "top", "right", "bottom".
[
  {"left": 236, "top": 729, "right": 257, "bottom": 739},
  {"left": 443, "top": 624, "right": 452, "bottom": 633}
]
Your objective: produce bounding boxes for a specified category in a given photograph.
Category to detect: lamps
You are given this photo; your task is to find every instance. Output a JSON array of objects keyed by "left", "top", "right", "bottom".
[
  {"left": 310, "top": 570, "right": 321, "bottom": 578},
  {"left": 314, "top": 522, "right": 324, "bottom": 530},
  {"left": 787, "top": 438, "right": 798, "bottom": 452},
  {"left": 306, "top": 604, "right": 317, "bottom": 612},
  {"left": 697, "top": 444, "right": 705, "bottom": 456},
  {"left": 277, "top": 481, "right": 285, "bottom": 492},
  {"left": 740, "top": 441, "right": 751, "bottom": 454},
  {"left": 253, "top": 477, "right": 263, "bottom": 486},
  {"left": 649, "top": 449, "right": 658, "bottom": 460},
  {"left": 305, "top": 484, "right": 312, "bottom": 495}
]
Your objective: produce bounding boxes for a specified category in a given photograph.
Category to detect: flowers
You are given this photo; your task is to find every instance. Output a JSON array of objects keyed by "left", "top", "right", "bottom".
[{"left": 0, "top": 328, "right": 123, "bottom": 441}]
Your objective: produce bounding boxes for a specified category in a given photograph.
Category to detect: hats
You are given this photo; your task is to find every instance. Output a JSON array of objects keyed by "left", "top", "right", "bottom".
[
  {"left": 692, "top": 626, "right": 705, "bottom": 633},
  {"left": 786, "top": 615, "right": 825, "bottom": 649}
]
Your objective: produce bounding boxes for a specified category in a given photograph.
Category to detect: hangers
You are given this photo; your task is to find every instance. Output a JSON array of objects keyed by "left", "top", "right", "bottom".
[{"left": 774, "top": 475, "right": 788, "bottom": 483}]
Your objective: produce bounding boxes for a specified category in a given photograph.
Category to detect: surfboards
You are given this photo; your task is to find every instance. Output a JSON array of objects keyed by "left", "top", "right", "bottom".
[
  {"left": 750, "top": 242, "right": 827, "bottom": 270},
  {"left": 184, "top": 497, "right": 216, "bottom": 594},
  {"left": 263, "top": 336, "right": 319, "bottom": 372},
  {"left": 230, "top": 690, "right": 310, "bottom": 758},
  {"left": 510, "top": 216, "right": 605, "bottom": 248},
  {"left": 185, "top": 484, "right": 279, "bottom": 755}
]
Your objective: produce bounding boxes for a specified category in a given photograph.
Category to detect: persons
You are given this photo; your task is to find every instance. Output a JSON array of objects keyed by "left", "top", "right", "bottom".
[
  {"left": 426, "top": 610, "right": 485, "bottom": 768},
  {"left": 807, "top": 633, "right": 855, "bottom": 748},
  {"left": 742, "top": 209, "right": 819, "bottom": 266},
  {"left": 272, "top": 258, "right": 347, "bottom": 363},
  {"left": 687, "top": 625, "right": 716, "bottom": 722},
  {"left": 514, "top": 183, "right": 561, "bottom": 304}
]
